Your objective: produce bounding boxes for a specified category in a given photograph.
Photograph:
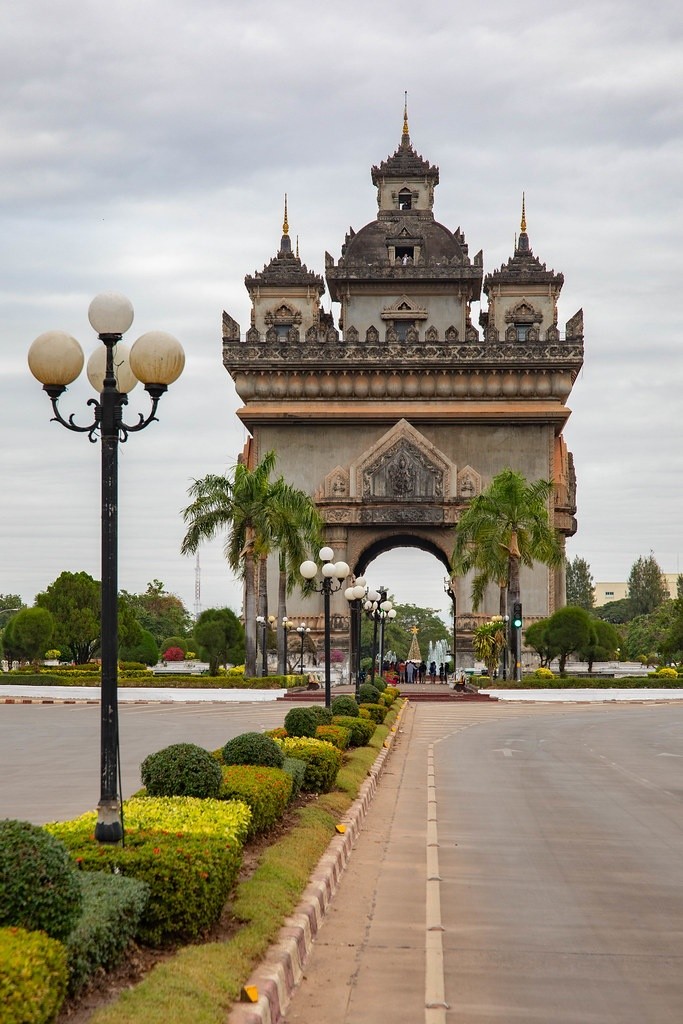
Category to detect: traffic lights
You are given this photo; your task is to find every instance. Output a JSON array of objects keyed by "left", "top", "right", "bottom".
[
  {"left": 514, "top": 601, "right": 522, "bottom": 628},
  {"left": 378, "top": 589, "right": 388, "bottom": 609}
]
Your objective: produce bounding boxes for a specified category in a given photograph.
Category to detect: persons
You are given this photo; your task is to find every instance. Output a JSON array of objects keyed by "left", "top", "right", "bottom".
[{"left": 382, "top": 660, "right": 449, "bottom": 684}]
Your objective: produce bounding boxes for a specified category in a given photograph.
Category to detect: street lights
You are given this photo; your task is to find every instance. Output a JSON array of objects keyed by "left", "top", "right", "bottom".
[
  {"left": 24, "top": 288, "right": 185, "bottom": 847},
  {"left": 295, "top": 622, "right": 311, "bottom": 676},
  {"left": 281, "top": 617, "right": 294, "bottom": 676},
  {"left": 343, "top": 576, "right": 366, "bottom": 707},
  {"left": 363, "top": 589, "right": 387, "bottom": 687},
  {"left": 378, "top": 599, "right": 397, "bottom": 676},
  {"left": 299, "top": 546, "right": 350, "bottom": 711},
  {"left": 255, "top": 616, "right": 275, "bottom": 677}
]
